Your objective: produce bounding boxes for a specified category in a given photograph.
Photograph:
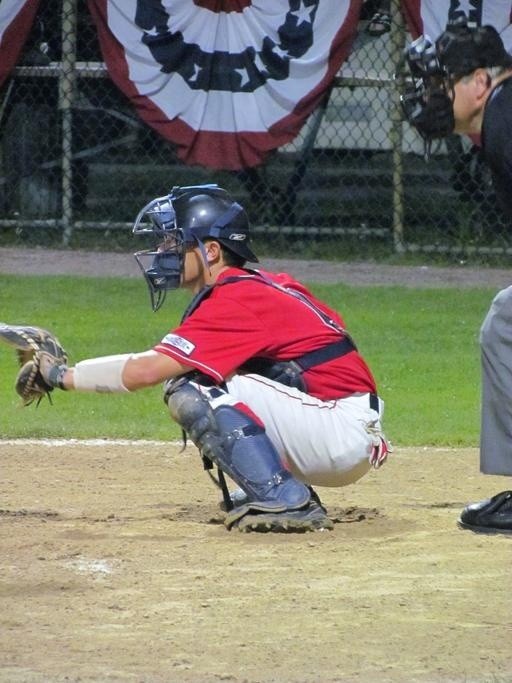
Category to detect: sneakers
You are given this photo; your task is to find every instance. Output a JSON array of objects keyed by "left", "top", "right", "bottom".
[
  {"left": 220, "top": 492, "right": 248, "bottom": 512},
  {"left": 231, "top": 502, "right": 334, "bottom": 536}
]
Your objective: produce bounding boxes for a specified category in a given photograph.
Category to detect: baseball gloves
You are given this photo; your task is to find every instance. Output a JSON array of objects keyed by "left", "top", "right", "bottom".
[{"left": 0, "top": 325, "right": 68, "bottom": 408}]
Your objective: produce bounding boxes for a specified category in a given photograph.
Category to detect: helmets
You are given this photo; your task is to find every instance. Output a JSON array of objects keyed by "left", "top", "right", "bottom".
[
  {"left": 133, "top": 183, "right": 258, "bottom": 313},
  {"left": 394, "top": 20, "right": 512, "bottom": 162}
]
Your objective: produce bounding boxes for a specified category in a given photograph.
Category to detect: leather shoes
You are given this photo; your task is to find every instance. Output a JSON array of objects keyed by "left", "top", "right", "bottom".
[{"left": 458, "top": 490, "right": 512, "bottom": 532}]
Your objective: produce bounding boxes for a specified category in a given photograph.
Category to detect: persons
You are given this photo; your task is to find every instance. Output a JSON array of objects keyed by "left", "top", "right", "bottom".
[
  {"left": 395, "top": 22, "right": 511, "bottom": 535},
  {"left": 0, "top": 178, "right": 389, "bottom": 532}
]
